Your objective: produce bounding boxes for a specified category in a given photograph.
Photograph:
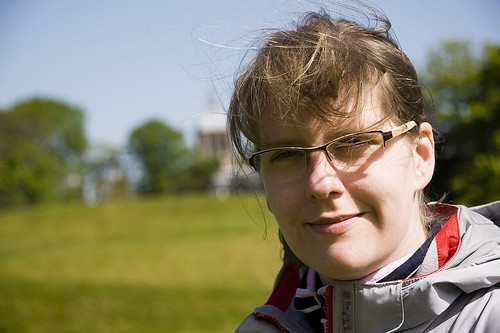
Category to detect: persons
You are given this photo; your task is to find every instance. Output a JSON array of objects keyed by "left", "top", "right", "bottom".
[{"left": 226, "top": 9, "right": 500, "bottom": 333}]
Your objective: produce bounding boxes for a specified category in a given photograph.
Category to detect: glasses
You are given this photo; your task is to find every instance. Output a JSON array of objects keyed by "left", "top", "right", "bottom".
[{"left": 248, "top": 122, "right": 418, "bottom": 184}]
